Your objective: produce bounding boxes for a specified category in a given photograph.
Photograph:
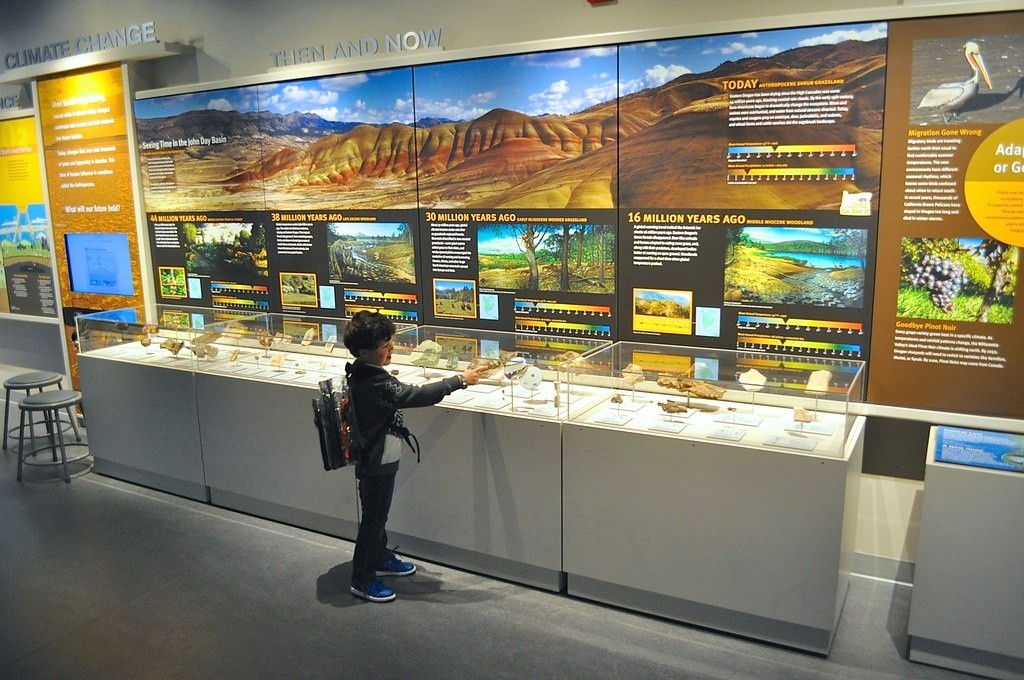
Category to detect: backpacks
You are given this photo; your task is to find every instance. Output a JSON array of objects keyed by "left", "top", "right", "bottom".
[{"left": 311, "top": 370, "right": 422, "bottom": 470}]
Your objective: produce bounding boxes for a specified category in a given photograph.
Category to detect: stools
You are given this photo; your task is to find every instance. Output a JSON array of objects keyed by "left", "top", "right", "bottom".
[
  {"left": 16, "top": 389, "right": 90, "bottom": 484},
  {"left": 3, "top": 371, "right": 81, "bottom": 450}
]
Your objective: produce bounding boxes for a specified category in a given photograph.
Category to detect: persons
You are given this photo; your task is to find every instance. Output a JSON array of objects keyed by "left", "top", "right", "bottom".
[{"left": 343, "top": 309, "right": 488, "bottom": 602}]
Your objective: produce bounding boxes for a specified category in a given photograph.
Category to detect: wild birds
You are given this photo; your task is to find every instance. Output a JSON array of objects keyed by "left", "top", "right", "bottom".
[{"left": 916, "top": 41, "right": 993, "bottom": 123}]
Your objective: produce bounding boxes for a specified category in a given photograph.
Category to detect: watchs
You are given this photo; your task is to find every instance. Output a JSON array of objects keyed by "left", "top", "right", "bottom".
[{"left": 459, "top": 373, "right": 468, "bottom": 389}]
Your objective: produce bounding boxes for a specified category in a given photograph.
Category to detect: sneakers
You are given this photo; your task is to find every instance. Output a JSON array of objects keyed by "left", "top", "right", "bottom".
[
  {"left": 375, "top": 545, "right": 417, "bottom": 576},
  {"left": 349, "top": 576, "right": 397, "bottom": 602}
]
